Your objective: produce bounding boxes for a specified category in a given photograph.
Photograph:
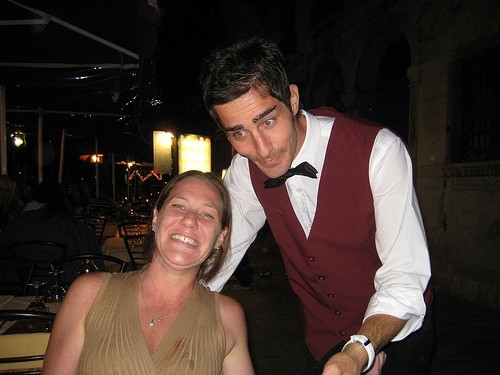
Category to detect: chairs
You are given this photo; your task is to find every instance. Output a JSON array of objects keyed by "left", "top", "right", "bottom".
[
  {"left": 77, "top": 217, "right": 107, "bottom": 246},
  {"left": 6, "top": 240, "right": 126, "bottom": 297},
  {"left": 117, "top": 217, "right": 147, "bottom": 237},
  {"left": 124, "top": 234, "right": 147, "bottom": 270},
  {"left": 0, "top": 310, "right": 55, "bottom": 375}
]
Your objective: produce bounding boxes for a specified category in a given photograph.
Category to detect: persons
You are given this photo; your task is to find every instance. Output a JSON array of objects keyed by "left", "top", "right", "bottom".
[
  {"left": 41, "top": 170, "right": 387, "bottom": 375},
  {"left": 197, "top": 39, "right": 433, "bottom": 375},
  {"left": 0, "top": 169, "right": 107, "bottom": 297}
]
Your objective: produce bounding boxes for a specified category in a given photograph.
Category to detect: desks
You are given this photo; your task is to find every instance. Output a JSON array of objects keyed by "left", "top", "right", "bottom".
[{"left": 102, "top": 238, "right": 145, "bottom": 271}]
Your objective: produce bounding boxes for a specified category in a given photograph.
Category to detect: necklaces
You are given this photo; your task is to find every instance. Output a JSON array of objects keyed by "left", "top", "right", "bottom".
[{"left": 139, "top": 274, "right": 195, "bottom": 329}]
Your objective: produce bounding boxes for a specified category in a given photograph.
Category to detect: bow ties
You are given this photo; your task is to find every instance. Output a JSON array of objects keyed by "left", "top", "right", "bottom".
[{"left": 263, "top": 162, "right": 318, "bottom": 188}]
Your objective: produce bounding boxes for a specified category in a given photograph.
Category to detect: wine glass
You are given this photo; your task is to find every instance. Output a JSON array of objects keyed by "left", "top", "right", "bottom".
[
  {"left": 46, "top": 269, "right": 67, "bottom": 302},
  {"left": 25, "top": 280, "right": 49, "bottom": 312},
  {"left": 120, "top": 195, "right": 145, "bottom": 247}
]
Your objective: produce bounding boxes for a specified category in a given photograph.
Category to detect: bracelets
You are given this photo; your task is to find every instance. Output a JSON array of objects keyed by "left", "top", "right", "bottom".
[{"left": 341, "top": 334, "right": 376, "bottom": 373}]
